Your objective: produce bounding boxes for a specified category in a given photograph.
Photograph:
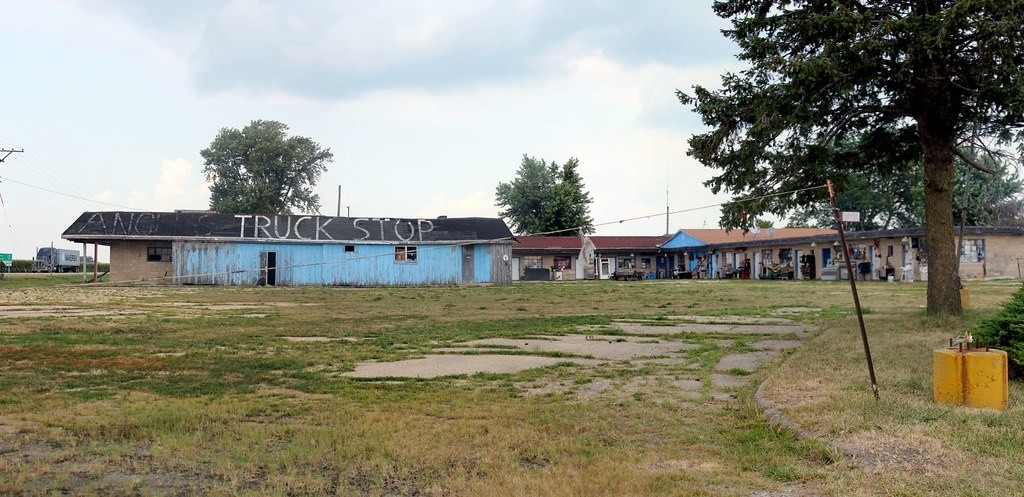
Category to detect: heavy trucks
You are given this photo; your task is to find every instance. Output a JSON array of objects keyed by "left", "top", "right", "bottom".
[{"left": 32, "top": 246, "right": 80, "bottom": 272}]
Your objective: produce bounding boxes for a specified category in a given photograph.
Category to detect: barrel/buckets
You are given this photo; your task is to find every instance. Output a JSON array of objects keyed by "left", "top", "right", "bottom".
[{"left": 888, "top": 274, "right": 894, "bottom": 282}]
